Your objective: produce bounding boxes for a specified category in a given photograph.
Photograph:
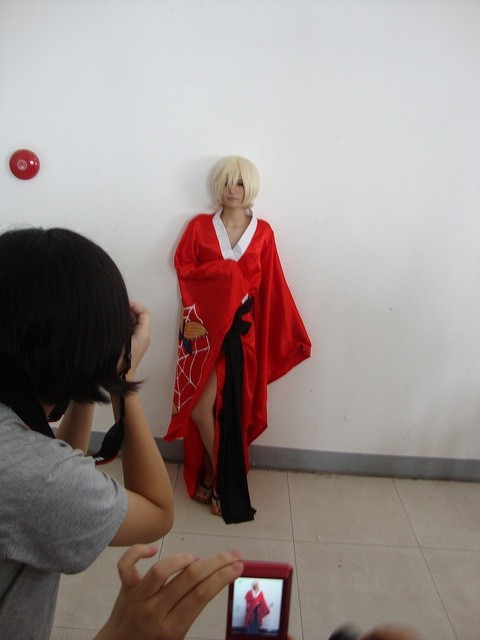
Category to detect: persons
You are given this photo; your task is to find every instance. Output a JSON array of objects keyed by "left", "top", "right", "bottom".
[
  {"left": 0, "top": 225, "right": 175, "bottom": 640},
  {"left": 244, "top": 582, "right": 270, "bottom": 629},
  {"left": 162, "top": 156, "right": 311, "bottom": 525},
  {"left": 93, "top": 543, "right": 417, "bottom": 640}
]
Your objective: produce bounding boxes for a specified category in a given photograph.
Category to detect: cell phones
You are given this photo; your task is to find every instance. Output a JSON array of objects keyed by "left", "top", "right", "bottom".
[{"left": 226, "top": 560, "right": 293, "bottom": 640}]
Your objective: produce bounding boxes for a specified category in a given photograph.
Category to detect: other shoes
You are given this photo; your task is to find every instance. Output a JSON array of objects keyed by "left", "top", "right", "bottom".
[
  {"left": 211, "top": 494, "right": 222, "bottom": 515},
  {"left": 199, "top": 481, "right": 212, "bottom": 503}
]
